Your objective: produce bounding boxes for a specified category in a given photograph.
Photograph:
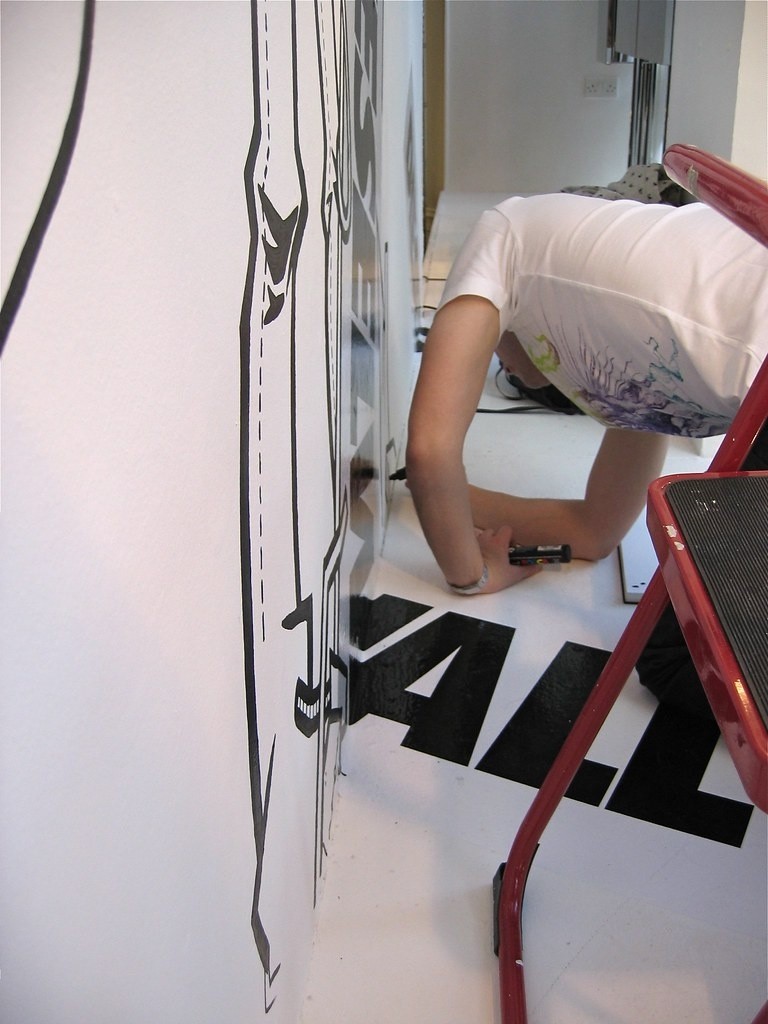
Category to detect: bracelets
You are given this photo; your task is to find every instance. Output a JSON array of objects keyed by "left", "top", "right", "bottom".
[{"left": 447, "top": 562, "right": 487, "bottom": 595}]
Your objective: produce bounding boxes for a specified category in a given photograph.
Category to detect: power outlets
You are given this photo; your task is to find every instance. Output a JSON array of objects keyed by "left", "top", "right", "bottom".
[{"left": 583, "top": 76, "right": 620, "bottom": 99}]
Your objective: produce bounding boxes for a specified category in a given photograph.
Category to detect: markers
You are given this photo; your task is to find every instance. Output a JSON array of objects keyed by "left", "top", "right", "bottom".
[{"left": 509, "top": 544, "right": 571, "bottom": 566}]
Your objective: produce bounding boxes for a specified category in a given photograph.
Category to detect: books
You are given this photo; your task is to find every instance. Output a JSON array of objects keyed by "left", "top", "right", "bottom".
[{"left": 617, "top": 505, "right": 659, "bottom": 604}]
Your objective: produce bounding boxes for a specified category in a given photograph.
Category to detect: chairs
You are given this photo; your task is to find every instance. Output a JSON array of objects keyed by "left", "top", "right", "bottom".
[{"left": 489, "top": 141, "right": 768, "bottom": 1024}]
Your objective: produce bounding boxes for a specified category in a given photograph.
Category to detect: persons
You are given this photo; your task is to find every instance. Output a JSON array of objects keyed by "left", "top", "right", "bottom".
[{"left": 406, "top": 194, "right": 768, "bottom": 596}]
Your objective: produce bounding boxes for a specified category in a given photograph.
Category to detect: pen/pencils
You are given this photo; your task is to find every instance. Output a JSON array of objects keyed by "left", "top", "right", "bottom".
[{"left": 389, "top": 467, "right": 407, "bottom": 480}]
